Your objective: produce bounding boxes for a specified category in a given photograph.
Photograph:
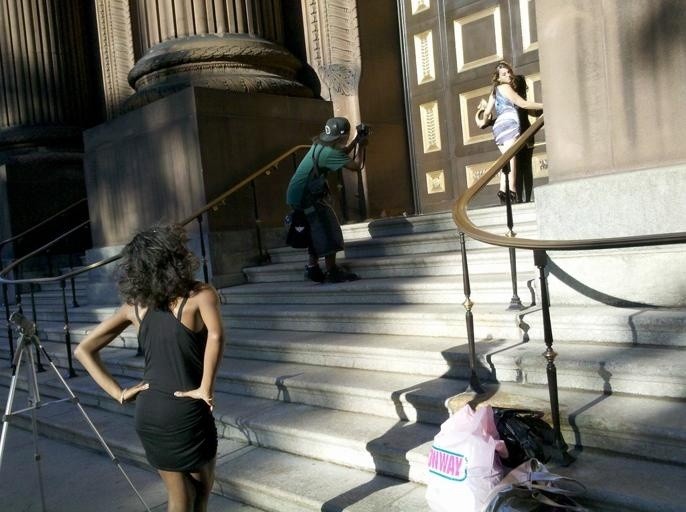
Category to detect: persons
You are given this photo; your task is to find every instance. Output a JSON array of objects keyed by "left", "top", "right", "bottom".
[
  {"left": 74, "top": 223, "right": 223, "bottom": 512},
  {"left": 477, "top": 62, "right": 543, "bottom": 205},
  {"left": 287, "top": 117, "right": 368, "bottom": 283}
]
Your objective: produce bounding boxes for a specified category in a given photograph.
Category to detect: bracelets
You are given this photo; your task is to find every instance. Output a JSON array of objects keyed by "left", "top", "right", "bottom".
[
  {"left": 119, "top": 388, "right": 127, "bottom": 405},
  {"left": 198, "top": 388, "right": 214, "bottom": 401}
]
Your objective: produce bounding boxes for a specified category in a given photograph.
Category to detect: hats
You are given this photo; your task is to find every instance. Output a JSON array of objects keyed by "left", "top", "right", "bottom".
[{"left": 319, "top": 116, "right": 351, "bottom": 143}]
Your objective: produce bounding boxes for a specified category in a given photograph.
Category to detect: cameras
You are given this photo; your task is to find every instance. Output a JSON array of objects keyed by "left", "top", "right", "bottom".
[{"left": 356, "top": 124, "right": 375, "bottom": 136}]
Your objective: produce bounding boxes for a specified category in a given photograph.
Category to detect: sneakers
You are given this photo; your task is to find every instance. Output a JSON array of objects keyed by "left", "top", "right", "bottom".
[
  {"left": 328, "top": 266, "right": 356, "bottom": 283},
  {"left": 302, "top": 263, "right": 326, "bottom": 282}
]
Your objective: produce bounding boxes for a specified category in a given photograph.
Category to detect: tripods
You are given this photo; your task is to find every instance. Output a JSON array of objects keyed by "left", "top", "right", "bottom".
[{"left": 0, "top": 306, "right": 153, "bottom": 512}]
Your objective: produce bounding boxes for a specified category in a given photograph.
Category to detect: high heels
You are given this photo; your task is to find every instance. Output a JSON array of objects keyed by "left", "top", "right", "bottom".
[{"left": 498, "top": 190, "right": 516, "bottom": 204}]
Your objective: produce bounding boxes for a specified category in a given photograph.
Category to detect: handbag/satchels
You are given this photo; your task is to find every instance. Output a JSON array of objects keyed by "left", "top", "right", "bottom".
[
  {"left": 308, "top": 172, "right": 332, "bottom": 195},
  {"left": 287, "top": 211, "right": 310, "bottom": 249},
  {"left": 482, "top": 457, "right": 590, "bottom": 512},
  {"left": 494, "top": 407, "right": 554, "bottom": 466}
]
